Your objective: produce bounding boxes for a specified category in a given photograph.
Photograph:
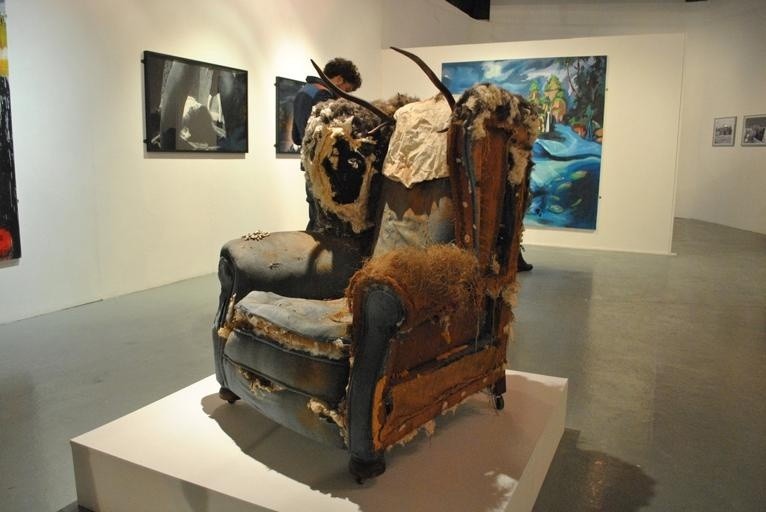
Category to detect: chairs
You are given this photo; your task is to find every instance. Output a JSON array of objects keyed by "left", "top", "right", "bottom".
[{"left": 211, "top": 83, "right": 541, "bottom": 485}]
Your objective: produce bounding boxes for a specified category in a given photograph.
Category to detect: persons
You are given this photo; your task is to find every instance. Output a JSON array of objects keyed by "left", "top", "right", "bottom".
[{"left": 287, "top": 57, "right": 363, "bottom": 235}]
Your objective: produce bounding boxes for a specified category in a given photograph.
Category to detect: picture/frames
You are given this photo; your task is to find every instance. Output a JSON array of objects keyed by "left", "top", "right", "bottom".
[
  {"left": 275, "top": 76, "right": 307, "bottom": 154},
  {"left": 141, "top": 50, "right": 249, "bottom": 154},
  {"left": 740, "top": 113, "right": 766, "bottom": 146},
  {"left": 711, "top": 116, "right": 737, "bottom": 146}
]
